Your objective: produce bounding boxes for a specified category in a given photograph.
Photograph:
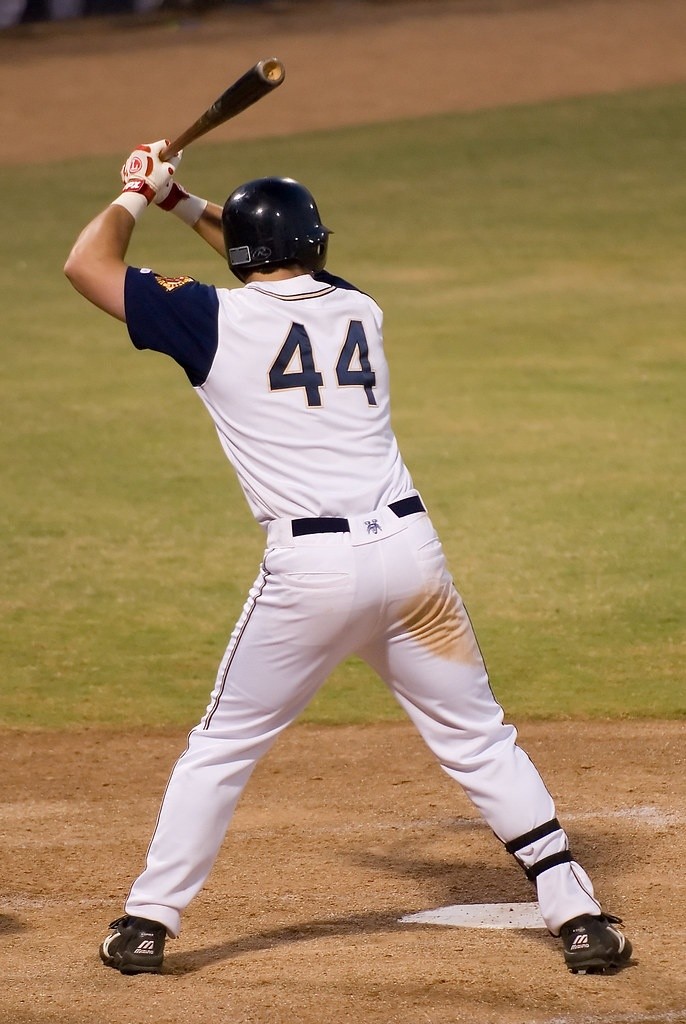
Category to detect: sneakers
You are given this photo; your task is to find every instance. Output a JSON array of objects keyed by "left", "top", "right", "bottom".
[
  {"left": 99, "top": 914, "right": 167, "bottom": 974},
  {"left": 559, "top": 914, "right": 633, "bottom": 974}
]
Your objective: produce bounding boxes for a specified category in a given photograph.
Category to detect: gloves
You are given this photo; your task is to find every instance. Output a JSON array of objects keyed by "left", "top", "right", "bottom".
[{"left": 109, "top": 139, "right": 208, "bottom": 228}]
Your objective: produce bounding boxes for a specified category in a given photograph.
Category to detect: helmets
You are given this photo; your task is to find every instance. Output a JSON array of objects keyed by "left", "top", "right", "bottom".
[{"left": 220, "top": 176, "right": 334, "bottom": 271}]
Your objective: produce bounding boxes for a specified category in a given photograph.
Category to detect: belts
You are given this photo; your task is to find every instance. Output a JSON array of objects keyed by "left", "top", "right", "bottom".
[{"left": 291, "top": 495, "right": 427, "bottom": 537}]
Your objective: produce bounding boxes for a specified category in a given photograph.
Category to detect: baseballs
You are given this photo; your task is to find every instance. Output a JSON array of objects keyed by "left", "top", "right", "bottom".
[{"left": 156, "top": 58, "right": 287, "bottom": 162}]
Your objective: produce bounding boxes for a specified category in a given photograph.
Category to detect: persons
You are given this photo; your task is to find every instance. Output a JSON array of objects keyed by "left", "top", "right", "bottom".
[{"left": 63, "top": 140, "right": 632, "bottom": 976}]
